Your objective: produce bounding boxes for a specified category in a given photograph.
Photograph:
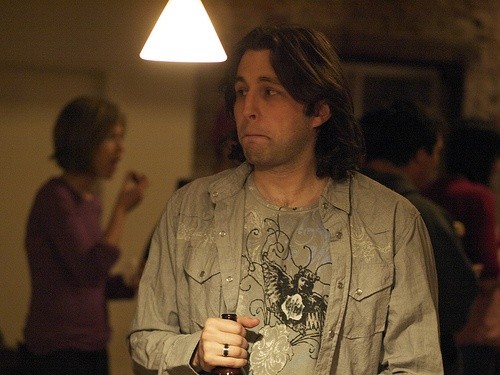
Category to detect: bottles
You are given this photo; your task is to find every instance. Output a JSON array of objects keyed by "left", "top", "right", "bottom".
[{"left": 208, "top": 313, "right": 247, "bottom": 375}]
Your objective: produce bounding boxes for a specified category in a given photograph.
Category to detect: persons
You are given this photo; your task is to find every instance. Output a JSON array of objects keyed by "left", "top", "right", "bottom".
[
  {"left": 126, "top": 24, "right": 444, "bottom": 375},
  {"left": 216, "top": 97, "right": 500, "bottom": 375},
  {"left": 17, "top": 95, "right": 151, "bottom": 375}
]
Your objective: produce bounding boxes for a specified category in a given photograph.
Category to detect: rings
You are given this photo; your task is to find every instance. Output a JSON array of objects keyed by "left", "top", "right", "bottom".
[{"left": 224, "top": 344, "right": 229, "bottom": 356}]
